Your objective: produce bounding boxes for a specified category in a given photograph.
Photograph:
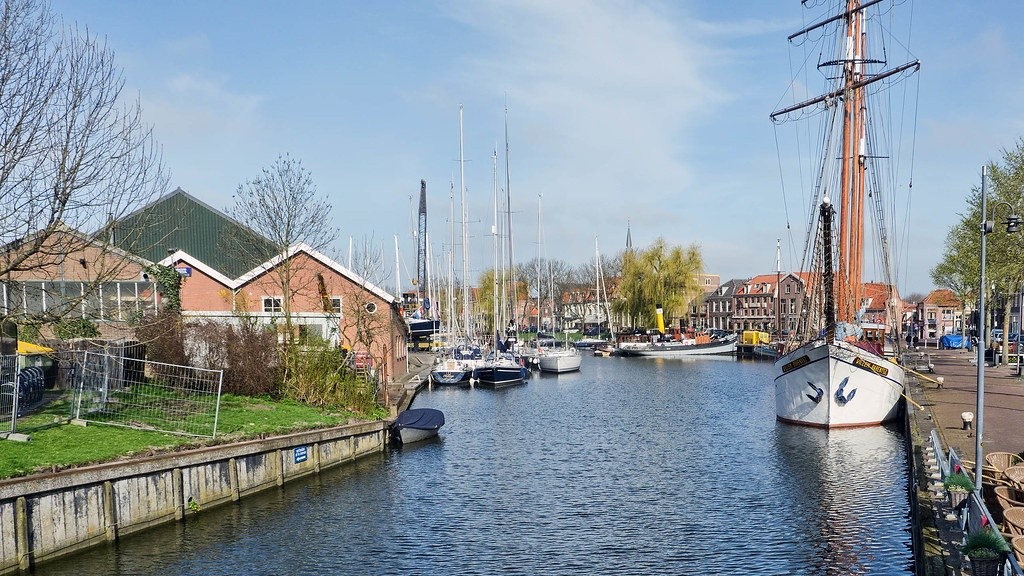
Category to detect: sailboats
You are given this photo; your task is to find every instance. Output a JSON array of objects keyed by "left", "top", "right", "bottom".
[
  {"left": 346, "top": 104, "right": 621, "bottom": 390},
  {"left": 768, "top": 0, "right": 906, "bottom": 427},
  {"left": 753, "top": 240, "right": 785, "bottom": 357}
]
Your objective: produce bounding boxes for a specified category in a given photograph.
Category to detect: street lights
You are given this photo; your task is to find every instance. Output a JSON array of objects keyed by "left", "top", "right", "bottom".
[{"left": 974, "top": 196, "right": 1022, "bottom": 490}]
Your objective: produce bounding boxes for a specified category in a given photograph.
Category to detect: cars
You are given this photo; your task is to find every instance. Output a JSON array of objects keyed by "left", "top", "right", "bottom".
[
  {"left": 537, "top": 328, "right": 562, "bottom": 338},
  {"left": 991, "top": 327, "right": 1024, "bottom": 347},
  {"left": 618, "top": 325, "right": 728, "bottom": 343},
  {"left": 523, "top": 326, "right": 537, "bottom": 332},
  {"left": 583, "top": 328, "right": 610, "bottom": 336}
]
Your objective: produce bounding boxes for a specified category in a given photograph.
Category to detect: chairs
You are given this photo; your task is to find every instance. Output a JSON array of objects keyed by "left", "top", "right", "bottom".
[{"left": 961, "top": 450, "right": 1024, "bottom": 570}]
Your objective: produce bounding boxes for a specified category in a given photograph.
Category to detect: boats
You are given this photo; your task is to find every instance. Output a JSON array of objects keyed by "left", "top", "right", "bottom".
[{"left": 393, "top": 407, "right": 445, "bottom": 444}]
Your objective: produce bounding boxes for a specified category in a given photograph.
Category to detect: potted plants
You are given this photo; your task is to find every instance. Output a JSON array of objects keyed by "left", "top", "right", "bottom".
[
  {"left": 958, "top": 526, "right": 1013, "bottom": 576},
  {"left": 943, "top": 472, "right": 977, "bottom": 509}
]
